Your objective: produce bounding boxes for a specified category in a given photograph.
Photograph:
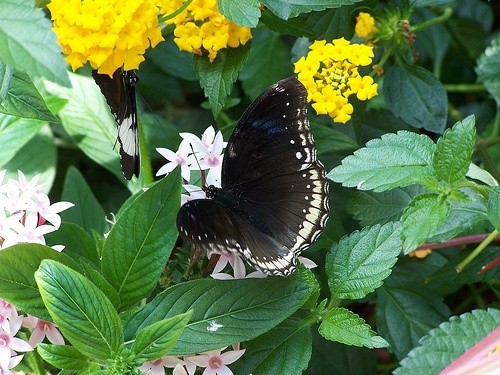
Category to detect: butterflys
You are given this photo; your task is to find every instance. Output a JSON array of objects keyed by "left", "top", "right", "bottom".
[
  {"left": 176, "top": 76, "right": 332, "bottom": 278},
  {"left": 90, "top": 64, "right": 165, "bottom": 181}
]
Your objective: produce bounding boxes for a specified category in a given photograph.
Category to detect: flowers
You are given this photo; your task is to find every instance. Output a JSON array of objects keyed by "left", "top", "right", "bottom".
[{"left": 0, "top": 0, "right": 499, "bottom": 375}]
